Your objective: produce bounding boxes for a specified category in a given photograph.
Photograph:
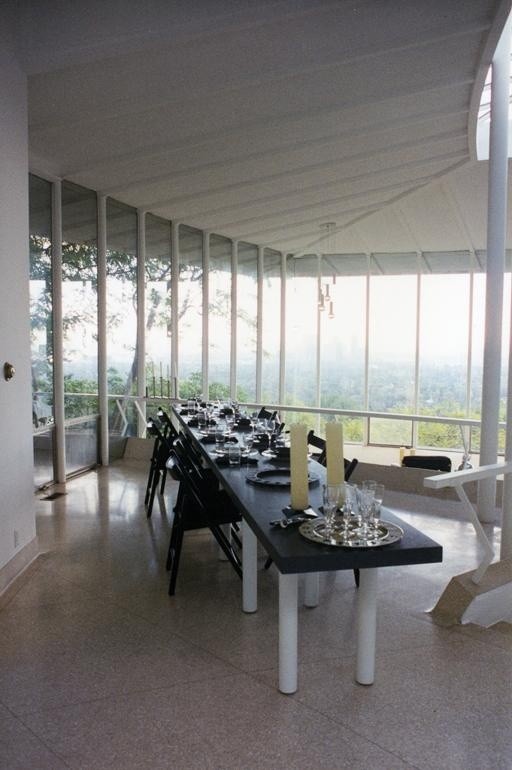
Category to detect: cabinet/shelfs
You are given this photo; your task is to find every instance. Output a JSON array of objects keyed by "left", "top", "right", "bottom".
[{"left": 400, "top": 445, "right": 416, "bottom": 461}]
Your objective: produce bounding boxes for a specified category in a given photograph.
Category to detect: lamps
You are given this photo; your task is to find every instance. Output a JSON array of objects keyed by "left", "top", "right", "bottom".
[
  {"left": 139, "top": 404, "right": 245, "bottom": 597},
  {"left": 257, "top": 408, "right": 358, "bottom": 481}
]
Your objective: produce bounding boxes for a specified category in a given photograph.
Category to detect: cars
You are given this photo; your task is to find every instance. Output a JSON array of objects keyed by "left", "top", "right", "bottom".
[{"left": 181, "top": 394, "right": 288, "bottom": 466}]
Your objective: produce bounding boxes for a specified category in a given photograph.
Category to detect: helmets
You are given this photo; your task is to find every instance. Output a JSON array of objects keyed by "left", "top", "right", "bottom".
[
  {"left": 261, "top": 449, "right": 313, "bottom": 461},
  {"left": 245, "top": 468, "right": 322, "bottom": 488},
  {"left": 298, "top": 518, "right": 404, "bottom": 548}
]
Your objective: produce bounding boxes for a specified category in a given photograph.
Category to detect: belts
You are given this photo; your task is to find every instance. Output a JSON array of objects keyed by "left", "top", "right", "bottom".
[{"left": 321, "top": 478, "right": 386, "bottom": 542}]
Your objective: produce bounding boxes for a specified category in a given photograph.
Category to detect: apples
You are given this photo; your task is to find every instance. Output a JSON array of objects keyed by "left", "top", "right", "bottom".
[
  {"left": 288, "top": 417, "right": 311, "bottom": 510},
  {"left": 324, "top": 415, "right": 346, "bottom": 502}
]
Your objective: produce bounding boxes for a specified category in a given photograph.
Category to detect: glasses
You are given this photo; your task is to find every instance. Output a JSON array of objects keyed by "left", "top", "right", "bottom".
[{"left": 314, "top": 202, "right": 340, "bottom": 322}]
